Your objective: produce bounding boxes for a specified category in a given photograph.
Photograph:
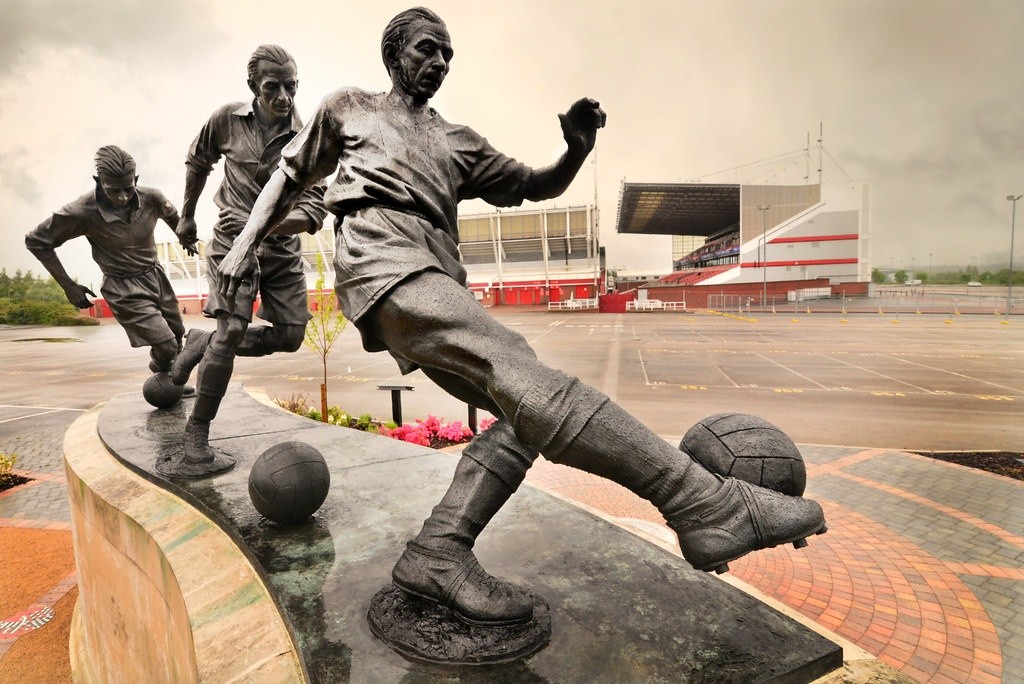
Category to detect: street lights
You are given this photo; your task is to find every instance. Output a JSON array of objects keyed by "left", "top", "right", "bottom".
[
  {"left": 757, "top": 204, "right": 772, "bottom": 309},
  {"left": 1005, "top": 194, "right": 1024, "bottom": 322}
]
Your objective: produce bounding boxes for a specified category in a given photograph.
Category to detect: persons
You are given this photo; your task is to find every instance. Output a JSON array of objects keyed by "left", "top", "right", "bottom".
[
  {"left": 172, "top": 42, "right": 328, "bottom": 467},
  {"left": 216, "top": 5, "right": 824, "bottom": 627},
  {"left": 25, "top": 145, "right": 201, "bottom": 396}
]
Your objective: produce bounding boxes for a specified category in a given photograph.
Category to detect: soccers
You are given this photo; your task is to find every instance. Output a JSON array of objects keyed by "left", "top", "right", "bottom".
[
  {"left": 248, "top": 441, "right": 330, "bottom": 525},
  {"left": 679, "top": 413, "right": 807, "bottom": 498},
  {"left": 143, "top": 372, "right": 183, "bottom": 409}
]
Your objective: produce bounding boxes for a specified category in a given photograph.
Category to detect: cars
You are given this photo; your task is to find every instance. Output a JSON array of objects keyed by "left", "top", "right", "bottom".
[{"left": 968, "top": 280, "right": 982, "bottom": 287}]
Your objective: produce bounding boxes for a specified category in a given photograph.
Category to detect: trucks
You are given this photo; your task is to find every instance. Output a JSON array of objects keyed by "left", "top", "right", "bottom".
[{"left": 905, "top": 280, "right": 922, "bottom": 286}]
